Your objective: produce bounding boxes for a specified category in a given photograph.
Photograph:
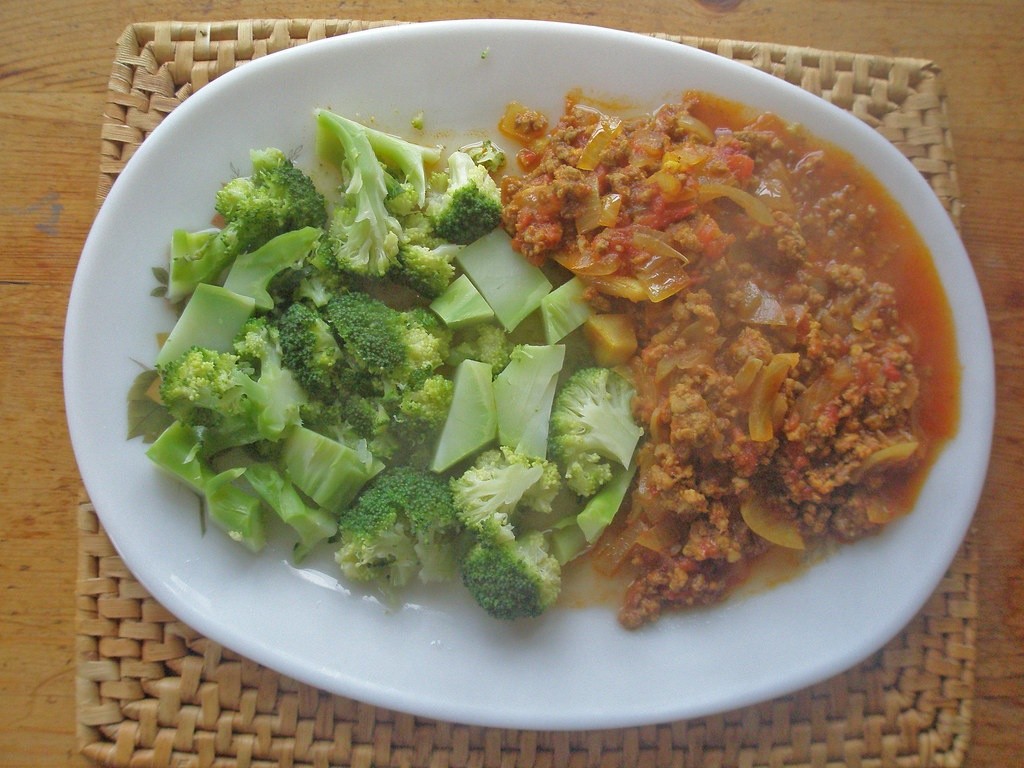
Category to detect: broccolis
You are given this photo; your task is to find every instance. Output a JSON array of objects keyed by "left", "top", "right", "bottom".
[{"left": 145, "top": 109, "right": 642, "bottom": 618}]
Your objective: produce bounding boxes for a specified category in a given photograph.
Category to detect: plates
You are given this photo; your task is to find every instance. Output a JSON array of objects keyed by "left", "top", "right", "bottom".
[{"left": 61, "top": 18, "right": 995, "bottom": 732}]
